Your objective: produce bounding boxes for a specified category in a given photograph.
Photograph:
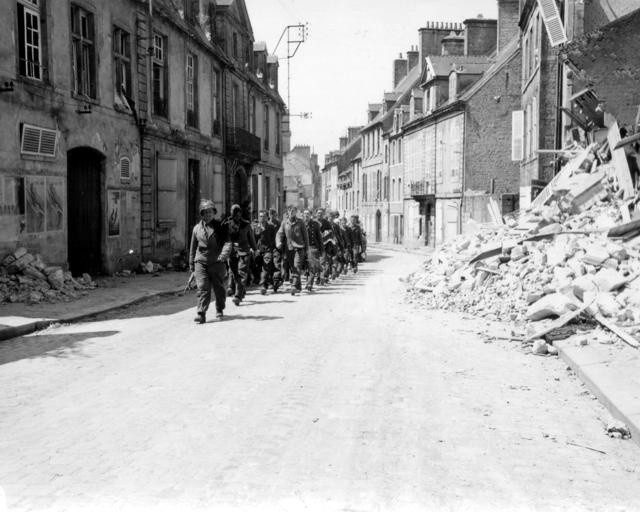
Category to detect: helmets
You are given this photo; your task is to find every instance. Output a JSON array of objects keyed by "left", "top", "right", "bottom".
[{"left": 197, "top": 198, "right": 217, "bottom": 216}]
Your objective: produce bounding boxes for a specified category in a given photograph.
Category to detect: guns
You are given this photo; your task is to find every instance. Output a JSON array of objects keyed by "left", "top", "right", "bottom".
[{"left": 185, "top": 272, "right": 195, "bottom": 293}]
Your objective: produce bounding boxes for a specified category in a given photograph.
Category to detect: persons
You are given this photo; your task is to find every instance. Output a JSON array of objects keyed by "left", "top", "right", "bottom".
[
  {"left": 222, "top": 204, "right": 257, "bottom": 306},
  {"left": 188, "top": 198, "right": 234, "bottom": 323},
  {"left": 216, "top": 206, "right": 366, "bottom": 296}
]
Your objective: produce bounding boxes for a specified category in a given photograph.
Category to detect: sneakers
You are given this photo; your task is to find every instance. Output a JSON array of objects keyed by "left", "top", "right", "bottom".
[{"left": 193, "top": 265, "right": 358, "bottom": 324}]
